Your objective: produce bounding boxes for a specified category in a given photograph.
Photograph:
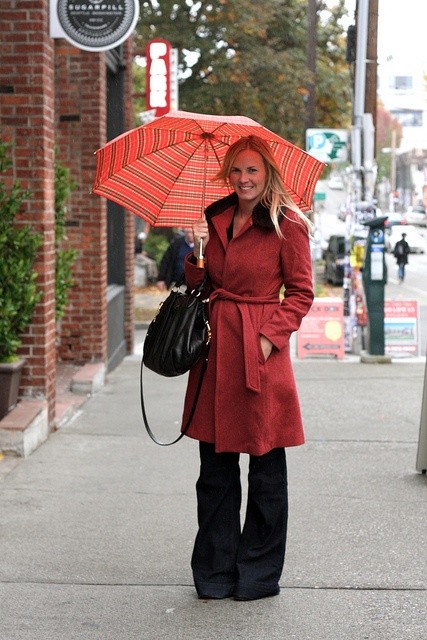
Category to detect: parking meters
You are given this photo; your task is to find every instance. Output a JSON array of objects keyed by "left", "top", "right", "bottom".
[{"left": 360, "top": 216, "right": 392, "bottom": 364}]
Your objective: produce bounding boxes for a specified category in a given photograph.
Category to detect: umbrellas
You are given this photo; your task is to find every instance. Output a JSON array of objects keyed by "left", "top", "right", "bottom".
[{"left": 88, "top": 115, "right": 327, "bottom": 270}]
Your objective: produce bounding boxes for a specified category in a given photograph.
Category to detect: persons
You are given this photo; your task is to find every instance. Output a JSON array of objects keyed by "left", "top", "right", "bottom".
[
  {"left": 157, "top": 232, "right": 200, "bottom": 290},
  {"left": 181, "top": 134, "right": 315, "bottom": 600},
  {"left": 396, "top": 234, "right": 411, "bottom": 281}
]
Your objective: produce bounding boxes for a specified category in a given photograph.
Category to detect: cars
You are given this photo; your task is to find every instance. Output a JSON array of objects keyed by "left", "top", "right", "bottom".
[
  {"left": 320, "top": 235, "right": 388, "bottom": 288},
  {"left": 385, "top": 224, "right": 425, "bottom": 254}
]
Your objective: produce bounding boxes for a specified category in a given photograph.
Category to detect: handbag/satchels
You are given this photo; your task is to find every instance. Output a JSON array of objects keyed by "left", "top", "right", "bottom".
[{"left": 140, "top": 271, "right": 211, "bottom": 377}]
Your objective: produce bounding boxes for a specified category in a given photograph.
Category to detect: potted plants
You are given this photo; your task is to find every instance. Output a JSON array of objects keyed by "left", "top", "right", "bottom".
[{"left": 0, "top": 131, "right": 45, "bottom": 418}]
